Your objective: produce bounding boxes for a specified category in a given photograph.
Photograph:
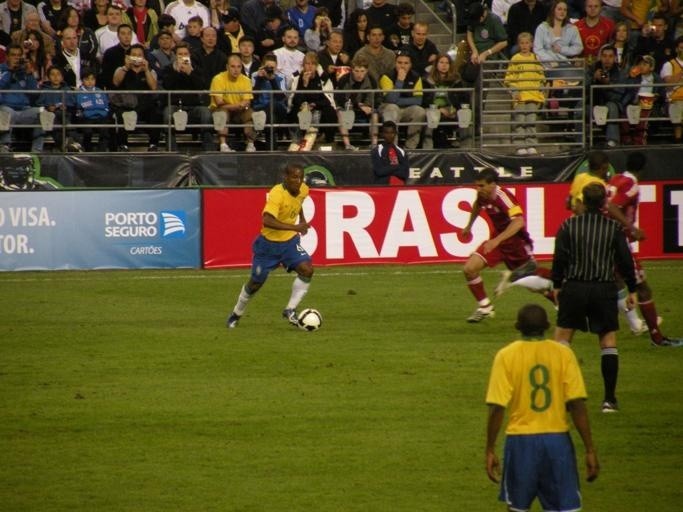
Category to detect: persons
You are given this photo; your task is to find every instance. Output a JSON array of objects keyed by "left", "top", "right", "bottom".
[
  {"left": 226, "top": 162, "right": 313, "bottom": 330},
  {"left": 509, "top": 153, "right": 682, "bottom": 347},
  {"left": 0, "top": 0, "right": 682, "bottom": 153},
  {"left": 484, "top": 304, "right": 599, "bottom": 511},
  {"left": 551, "top": 181, "right": 637, "bottom": 413},
  {"left": 370, "top": 121, "right": 409, "bottom": 187},
  {"left": 493, "top": 152, "right": 662, "bottom": 336},
  {"left": 458, "top": 167, "right": 554, "bottom": 322}
]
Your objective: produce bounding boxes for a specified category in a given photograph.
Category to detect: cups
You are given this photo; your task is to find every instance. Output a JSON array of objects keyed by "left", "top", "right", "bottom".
[{"left": 628, "top": 65, "right": 642, "bottom": 77}]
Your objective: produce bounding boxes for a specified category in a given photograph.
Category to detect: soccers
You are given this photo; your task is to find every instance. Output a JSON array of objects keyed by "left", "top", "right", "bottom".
[{"left": 298, "top": 308, "right": 322, "bottom": 332}]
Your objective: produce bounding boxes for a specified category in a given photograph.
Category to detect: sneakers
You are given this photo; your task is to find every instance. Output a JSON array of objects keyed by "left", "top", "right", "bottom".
[
  {"left": 631, "top": 317, "right": 661, "bottom": 335},
  {"left": 652, "top": 337, "right": 683, "bottom": 347},
  {"left": 516, "top": 148, "right": 537, "bottom": 156},
  {"left": 220, "top": 142, "right": 236, "bottom": 152},
  {"left": 227, "top": 311, "right": 240, "bottom": 328},
  {"left": 119, "top": 145, "right": 128, "bottom": 152},
  {"left": 282, "top": 309, "right": 299, "bottom": 326},
  {"left": 287, "top": 143, "right": 300, "bottom": 153},
  {"left": 601, "top": 401, "right": 619, "bottom": 413},
  {"left": 345, "top": 144, "right": 359, "bottom": 151},
  {"left": 246, "top": 142, "right": 256, "bottom": 152},
  {"left": 467, "top": 306, "right": 495, "bottom": 322}
]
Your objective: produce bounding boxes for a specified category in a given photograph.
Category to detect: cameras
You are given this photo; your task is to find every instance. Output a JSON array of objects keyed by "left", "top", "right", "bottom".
[
  {"left": 600, "top": 70, "right": 609, "bottom": 79},
  {"left": 651, "top": 26, "right": 657, "bottom": 32},
  {"left": 319, "top": 16, "right": 326, "bottom": 22},
  {"left": 265, "top": 66, "right": 274, "bottom": 75},
  {"left": 182, "top": 57, "right": 190, "bottom": 65},
  {"left": 131, "top": 56, "right": 143, "bottom": 66},
  {"left": 24, "top": 38, "right": 32, "bottom": 47}
]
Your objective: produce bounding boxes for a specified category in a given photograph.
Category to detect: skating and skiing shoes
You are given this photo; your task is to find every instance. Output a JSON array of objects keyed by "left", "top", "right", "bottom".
[{"left": 148, "top": 146, "right": 157, "bottom": 151}]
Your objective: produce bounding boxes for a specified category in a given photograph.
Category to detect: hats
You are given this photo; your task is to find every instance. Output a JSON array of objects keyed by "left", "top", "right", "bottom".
[
  {"left": 636, "top": 52, "right": 655, "bottom": 67},
  {"left": 220, "top": 7, "right": 239, "bottom": 21},
  {"left": 468, "top": 3, "right": 482, "bottom": 26}
]
